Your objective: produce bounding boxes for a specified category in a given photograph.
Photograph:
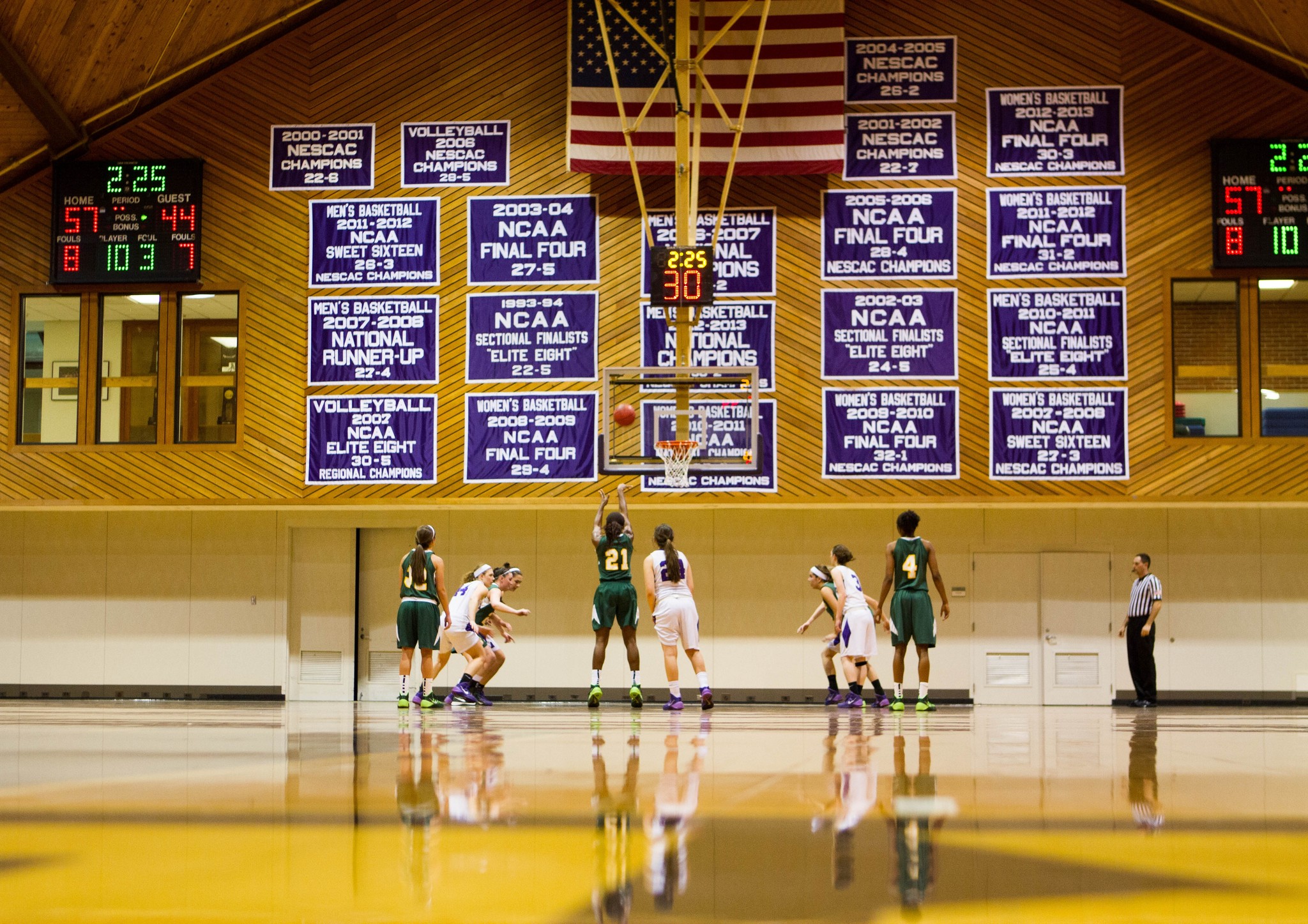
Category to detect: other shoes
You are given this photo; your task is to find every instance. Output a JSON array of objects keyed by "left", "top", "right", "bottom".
[
  {"left": 1127, "top": 700, "right": 1143, "bottom": 707},
  {"left": 1134, "top": 700, "right": 1158, "bottom": 708}
]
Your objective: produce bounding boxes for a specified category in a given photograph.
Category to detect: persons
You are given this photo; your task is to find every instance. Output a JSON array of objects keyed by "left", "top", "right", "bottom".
[
  {"left": 433, "top": 706, "right": 513, "bottom": 823},
  {"left": 797, "top": 544, "right": 889, "bottom": 708},
  {"left": 396, "top": 708, "right": 440, "bottom": 830},
  {"left": 413, "top": 563, "right": 530, "bottom": 706},
  {"left": 644, "top": 524, "right": 714, "bottom": 710},
  {"left": 810, "top": 707, "right": 878, "bottom": 888},
  {"left": 591, "top": 736, "right": 644, "bottom": 924},
  {"left": 640, "top": 712, "right": 713, "bottom": 913},
  {"left": 874, "top": 509, "right": 950, "bottom": 711},
  {"left": 1129, "top": 709, "right": 1162, "bottom": 837},
  {"left": 587, "top": 483, "right": 643, "bottom": 707},
  {"left": 1118, "top": 553, "right": 1162, "bottom": 708},
  {"left": 396, "top": 524, "right": 452, "bottom": 708},
  {"left": 876, "top": 713, "right": 943, "bottom": 924}
]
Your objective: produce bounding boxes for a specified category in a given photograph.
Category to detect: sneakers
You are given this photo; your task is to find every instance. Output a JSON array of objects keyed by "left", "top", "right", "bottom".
[
  {"left": 888, "top": 693, "right": 905, "bottom": 710},
  {"left": 699, "top": 685, "right": 714, "bottom": 710},
  {"left": 915, "top": 693, "right": 937, "bottom": 711},
  {"left": 451, "top": 695, "right": 477, "bottom": 706},
  {"left": 629, "top": 683, "right": 643, "bottom": 708},
  {"left": 396, "top": 690, "right": 409, "bottom": 708},
  {"left": 412, "top": 687, "right": 423, "bottom": 706},
  {"left": 474, "top": 691, "right": 493, "bottom": 706},
  {"left": 585, "top": 683, "right": 603, "bottom": 707},
  {"left": 870, "top": 692, "right": 889, "bottom": 708},
  {"left": 420, "top": 690, "right": 445, "bottom": 708},
  {"left": 824, "top": 687, "right": 843, "bottom": 706},
  {"left": 837, "top": 691, "right": 863, "bottom": 708},
  {"left": 444, "top": 693, "right": 454, "bottom": 705},
  {"left": 662, "top": 693, "right": 685, "bottom": 710},
  {"left": 451, "top": 681, "right": 478, "bottom": 702}
]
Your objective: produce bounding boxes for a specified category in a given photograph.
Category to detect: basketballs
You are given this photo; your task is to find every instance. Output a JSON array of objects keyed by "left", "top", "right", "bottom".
[{"left": 613, "top": 402, "right": 637, "bottom": 427}]
